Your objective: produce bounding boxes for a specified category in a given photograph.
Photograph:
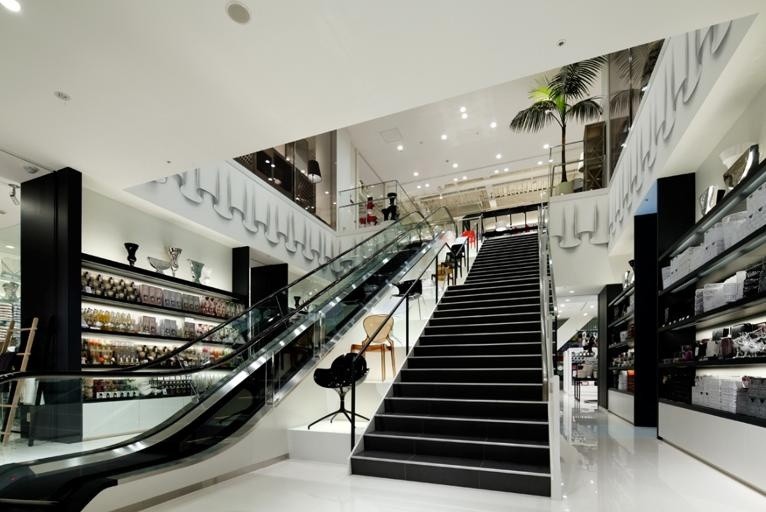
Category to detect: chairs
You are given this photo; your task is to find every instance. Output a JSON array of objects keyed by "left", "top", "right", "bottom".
[{"left": 307, "top": 229, "right": 476, "bottom": 429}]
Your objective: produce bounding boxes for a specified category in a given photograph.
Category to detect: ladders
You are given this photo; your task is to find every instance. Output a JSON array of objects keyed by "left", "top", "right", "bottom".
[{"left": 0, "top": 317, "right": 39, "bottom": 446}]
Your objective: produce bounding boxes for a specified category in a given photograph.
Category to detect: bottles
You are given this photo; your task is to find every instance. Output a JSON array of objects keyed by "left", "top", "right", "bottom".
[{"left": 82, "top": 308, "right": 139, "bottom": 364}]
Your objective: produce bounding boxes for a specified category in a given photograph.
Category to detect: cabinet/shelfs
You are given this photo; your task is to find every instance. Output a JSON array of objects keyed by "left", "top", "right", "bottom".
[
  {"left": 566, "top": 347, "right": 599, "bottom": 396},
  {"left": 81, "top": 252, "right": 245, "bottom": 441},
  {"left": 597, "top": 212, "right": 656, "bottom": 427},
  {"left": 656, "top": 160, "right": 766, "bottom": 495}
]
